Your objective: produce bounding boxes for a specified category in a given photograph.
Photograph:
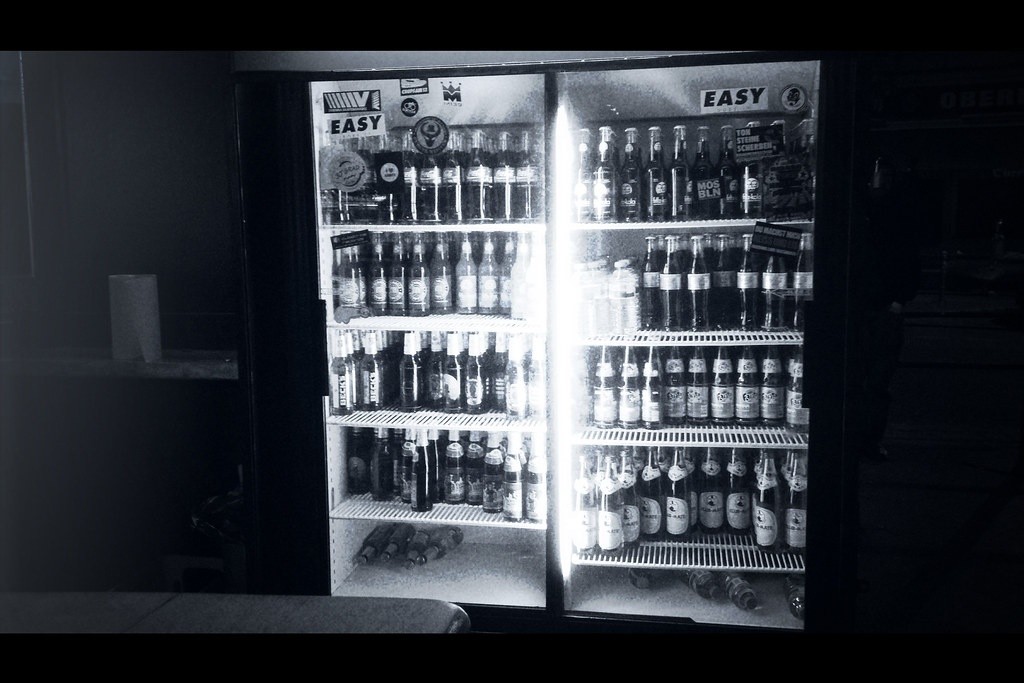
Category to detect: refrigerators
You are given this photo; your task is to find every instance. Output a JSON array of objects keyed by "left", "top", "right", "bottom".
[{"left": 310, "top": 60, "right": 819, "bottom": 629}]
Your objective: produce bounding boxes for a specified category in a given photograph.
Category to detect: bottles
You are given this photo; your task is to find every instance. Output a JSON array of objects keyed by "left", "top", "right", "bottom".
[
  {"left": 464, "top": 334, "right": 530, "bottom": 415},
  {"left": 662, "top": 347, "right": 735, "bottom": 428},
  {"left": 719, "top": 119, "right": 812, "bottom": 219},
  {"left": 573, "top": 128, "right": 616, "bottom": 223},
  {"left": 358, "top": 521, "right": 464, "bottom": 569},
  {"left": 580, "top": 445, "right": 807, "bottom": 622},
  {"left": 641, "top": 235, "right": 686, "bottom": 330},
  {"left": 321, "top": 130, "right": 403, "bottom": 223},
  {"left": 736, "top": 348, "right": 809, "bottom": 431},
  {"left": 688, "top": 234, "right": 737, "bottom": 331},
  {"left": 670, "top": 126, "right": 719, "bottom": 219},
  {"left": 335, "top": 232, "right": 387, "bottom": 316},
  {"left": 626, "top": 566, "right": 806, "bottom": 623},
  {"left": 618, "top": 128, "right": 668, "bottom": 222},
  {"left": 478, "top": 231, "right": 517, "bottom": 316},
  {"left": 738, "top": 234, "right": 813, "bottom": 330},
  {"left": 463, "top": 129, "right": 538, "bottom": 219},
  {"left": 347, "top": 428, "right": 538, "bottom": 521},
  {"left": 432, "top": 231, "right": 477, "bottom": 316},
  {"left": 593, "top": 347, "right": 662, "bottom": 429},
  {"left": 400, "top": 331, "right": 468, "bottom": 413},
  {"left": 329, "top": 330, "right": 401, "bottom": 416},
  {"left": 388, "top": 232, "right": 434, "bottom": 317},
  {"left": 584, "top": 256, "right": 642, "bottom": 333},
  {"left": 400, "top": 130, "right": 465, "bottom": 222}
]
